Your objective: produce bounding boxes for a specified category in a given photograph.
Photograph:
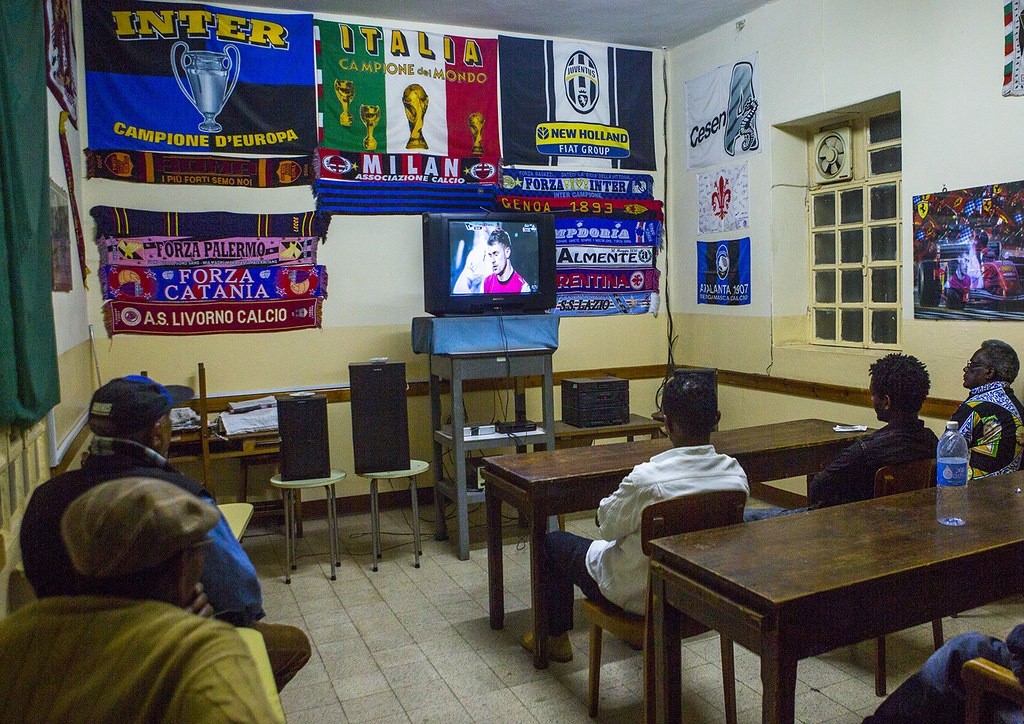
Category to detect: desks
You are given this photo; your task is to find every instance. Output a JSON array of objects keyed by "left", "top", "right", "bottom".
[
  {"left": 647, "top": 472, "right": 1024, "bottom": 724},
  {"left": 169, "top": 414, "right": 303, "bottom": 538},
  {"left": 483, "top": 419, "right": 890, "bottom": 671},
  {"left": 533, "top": 413, "right": 664, "bottom": 534},
  {"left": 15, "top": 502, "right": 254, "bottom": 580}
]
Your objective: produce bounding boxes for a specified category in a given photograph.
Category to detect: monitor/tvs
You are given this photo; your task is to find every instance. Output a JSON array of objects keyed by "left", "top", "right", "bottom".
[{"left": 422, "top": 212, "right": 556, "bottom": 318}]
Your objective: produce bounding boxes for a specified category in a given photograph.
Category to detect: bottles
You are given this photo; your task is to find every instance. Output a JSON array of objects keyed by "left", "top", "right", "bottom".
[{"left": 938, "top": 421, "right": 969, "bottom": 527}]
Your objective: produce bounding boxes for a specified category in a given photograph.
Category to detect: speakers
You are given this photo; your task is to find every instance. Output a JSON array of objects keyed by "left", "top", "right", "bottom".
[
  {"left": 276, "top": 393, "right": 330, "bottom": 482},
  {"left": 349, "top": 361, "right": 410, "bottom": 473},
  {"left": 675, "top": 367, "right": 720, "bottom": 431}
]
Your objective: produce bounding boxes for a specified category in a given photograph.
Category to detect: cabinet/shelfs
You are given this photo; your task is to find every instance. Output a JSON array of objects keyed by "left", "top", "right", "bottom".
[{"left": 430, "top": 348, "right": 556, "bottom": 559}]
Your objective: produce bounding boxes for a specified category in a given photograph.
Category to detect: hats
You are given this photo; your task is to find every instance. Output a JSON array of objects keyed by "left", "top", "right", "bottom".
[
  {"left": 60, "top": 476, "right": 217, "bottom": 579},
  {"left": 88, "top": 375, "right": 195, "bottom": 438}
]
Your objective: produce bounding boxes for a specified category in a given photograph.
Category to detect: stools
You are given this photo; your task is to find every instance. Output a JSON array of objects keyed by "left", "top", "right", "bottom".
[
  {"left": 357, "top": 459, "right": 430, "bottom": 572},
  {"left": 269, "top": 470, "right": 346, "bottom": 583}
]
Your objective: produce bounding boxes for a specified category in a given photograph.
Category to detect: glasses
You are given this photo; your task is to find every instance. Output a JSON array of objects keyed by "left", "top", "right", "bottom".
[
  {"left": 967, "top": 361, "right": 989, "bottom": 370},
  {"left": 185, "top": 536, "right": 214, "bottom": 551}
]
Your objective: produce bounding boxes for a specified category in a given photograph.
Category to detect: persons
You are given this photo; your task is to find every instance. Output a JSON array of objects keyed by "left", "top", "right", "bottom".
[
  {"left": 480, "top": 228, "right": 531, "bottom": 293},
  {"left": 744, "top": 353, "right": 939, "bottom": 523},
  {"left": 860, "top": 624, "right": 1024, "bottom": 724},
  {"left": 0, "top": 375, "right": 313, "bottom": 724},
  {"left": 946, "top": 339, "right": 1024, "bottom": 480},
  {"left": 941, "top": 231, "right": 996, "bottom": 310},
  {"left": 522, "top": 371, "right": 750, "bottom": 660}
]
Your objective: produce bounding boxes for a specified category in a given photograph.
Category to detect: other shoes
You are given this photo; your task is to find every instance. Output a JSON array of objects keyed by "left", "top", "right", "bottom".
[{"left": 521, "top": 632, "right": 573, "bottom": 663}]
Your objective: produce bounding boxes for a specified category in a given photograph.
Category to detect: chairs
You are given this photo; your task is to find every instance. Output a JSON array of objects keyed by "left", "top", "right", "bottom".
[
  {"left": 875, "top": 460, "right": 947, "bottom": 698},
  {"left": 582, "top": 491, "right": 739, "bottom": 724}
]
